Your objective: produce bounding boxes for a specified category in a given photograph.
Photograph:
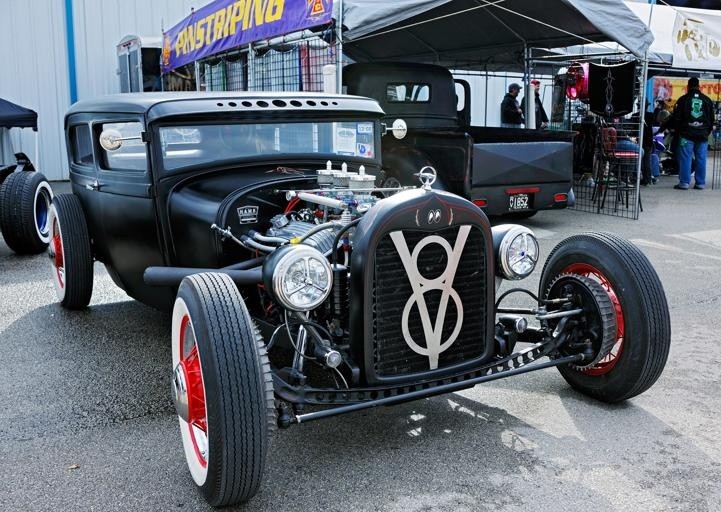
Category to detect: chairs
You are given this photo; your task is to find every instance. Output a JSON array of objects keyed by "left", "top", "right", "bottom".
[{"left": 590, "top": 128, "right": 642, "bottom": 213}]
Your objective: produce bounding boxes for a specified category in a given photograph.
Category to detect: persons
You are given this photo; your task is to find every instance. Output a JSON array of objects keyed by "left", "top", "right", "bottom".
[
  {"left": 652, "top": 99, "right": 672, "bottom": 140},
  {"left": 630, "top": 96, "right": 656, "bottom": 187},
  {"left": 672, "top": 78, "right": 715, "bottom": 189},
  {"left": 520, "top": 80, "right": 550, "bottom": 129},
  {"left": 502, "top": 83, "right": 526, "bottom": 130}
]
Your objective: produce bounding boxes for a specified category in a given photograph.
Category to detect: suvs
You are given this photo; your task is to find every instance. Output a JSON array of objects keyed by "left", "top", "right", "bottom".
[
  {"left": 1, "top": 98, "right": 54, "bottom": 256},
  {"left": 43, "top": 86, "right": 672, "bottom": 512}
]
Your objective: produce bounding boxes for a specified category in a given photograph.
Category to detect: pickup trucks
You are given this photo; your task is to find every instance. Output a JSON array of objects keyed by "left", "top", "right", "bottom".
[{"left": 343, "top": 61, "right": 578, "bottom": 223}]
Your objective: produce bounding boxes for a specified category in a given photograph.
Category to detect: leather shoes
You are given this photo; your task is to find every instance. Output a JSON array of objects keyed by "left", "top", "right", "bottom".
[{"left": 674, "top": 184, "right": 688, "bottom": 190}]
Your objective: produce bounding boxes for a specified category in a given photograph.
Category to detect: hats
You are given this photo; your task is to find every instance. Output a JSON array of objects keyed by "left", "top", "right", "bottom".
[
  {"left": 531, "top": 80, "right": 539, "bottom": 85},
  {"left": 508, "top": 83, "right": 522, "bottom": 89}
]
